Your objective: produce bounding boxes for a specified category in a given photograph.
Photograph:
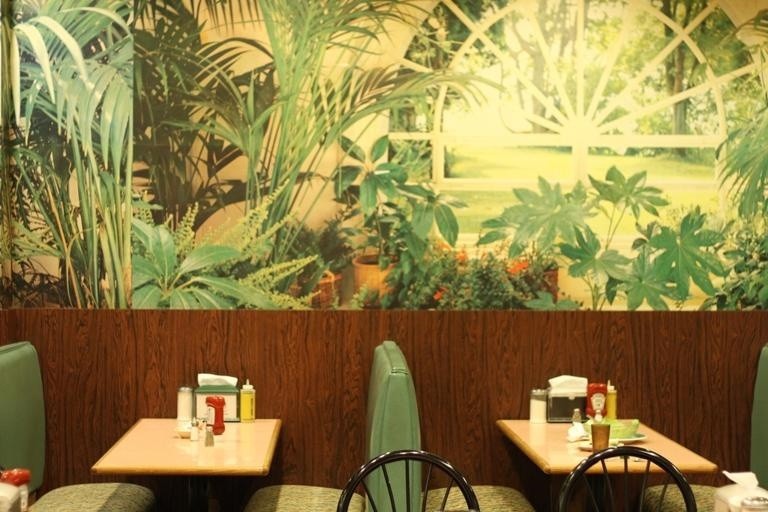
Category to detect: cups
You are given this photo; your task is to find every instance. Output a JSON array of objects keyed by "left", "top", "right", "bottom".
[{"left": 590, "top": 423, "right": 612, "bottom": 453}]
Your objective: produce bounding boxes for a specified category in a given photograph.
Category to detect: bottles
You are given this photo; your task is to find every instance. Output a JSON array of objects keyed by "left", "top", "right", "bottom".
[
  {"left": 528, "top": 388, "right": 547, "bottom": 424},
  {"left": 740, "top": 497, "right": 768, "bottom": 512},
  {"left": 571, "top": 408, "right": 583, "bottom": 424},
  {"left": 0, "top": 468, "right": 30, "bottom": 512},
  {"left": 189, "top": 416, "right": 214, "bottom": 447},
  {"left": 175, "top": 386, "right": 192, "bottom": 422},
  {"left": 205, "top": 396, "right": 226, "bottom": 436},
  {"left": 240, "top": 379, "right": 257, "bottom": 424}
]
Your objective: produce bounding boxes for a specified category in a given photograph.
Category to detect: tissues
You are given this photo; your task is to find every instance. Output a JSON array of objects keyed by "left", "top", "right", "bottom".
[
  {"left": 193, "top": 372, "right": 240, "bottom": 423},
  {"left": 545, "top": 373, "right": 590, "bottom": 424}
]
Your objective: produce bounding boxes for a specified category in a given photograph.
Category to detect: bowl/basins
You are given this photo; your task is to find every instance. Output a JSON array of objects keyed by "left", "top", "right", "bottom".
[
  {"left": 175, "top": 428, "right": 191, "bottom": 439},
  {"left": 584, "top": 420, "right": 640, "bottom": 437}
]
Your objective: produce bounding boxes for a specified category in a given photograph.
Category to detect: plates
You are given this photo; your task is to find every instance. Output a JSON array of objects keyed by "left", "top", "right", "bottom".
[{"left": 612, "top": 434, "right": 648, "bottom": 445}]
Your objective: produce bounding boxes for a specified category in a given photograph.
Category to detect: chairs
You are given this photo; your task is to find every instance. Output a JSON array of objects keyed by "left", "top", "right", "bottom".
[
  {"left": 556, "top": 444, "right": 706, "bottom": 511},
  {"left": 337, "top": 444, "right": 483, "bottom": 512}
]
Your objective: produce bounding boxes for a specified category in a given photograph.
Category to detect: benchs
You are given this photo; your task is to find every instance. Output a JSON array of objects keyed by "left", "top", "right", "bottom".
[
  {"left": 0, "top": 331, "right": 160, "bottom": 512},
  {"left": 641, "top": 345, "right": 768, "bottom": 511},
  {"left": 391, "top": 343, "right": 540, "bottom": 512},
  {"left": 244, "top": 341, "right": 399, "bottom": 511}
]
